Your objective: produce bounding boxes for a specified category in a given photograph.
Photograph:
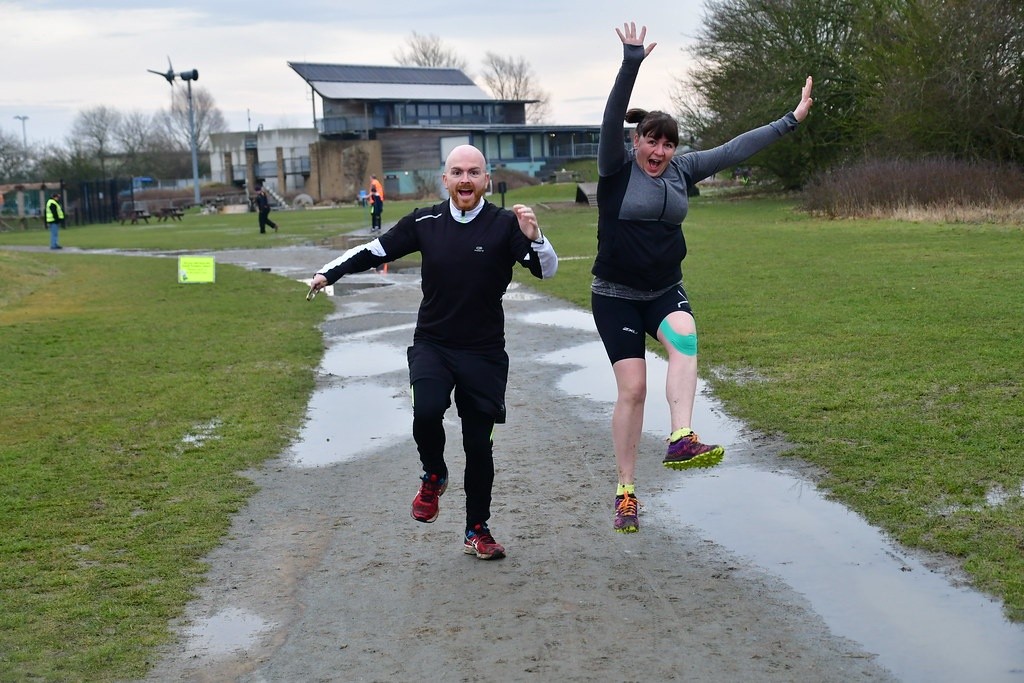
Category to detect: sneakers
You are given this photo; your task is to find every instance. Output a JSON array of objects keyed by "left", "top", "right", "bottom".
[
  {"left": 662, "top": 431, "right": 724, "bottom": 472},
  {"left": 614, "top": 492, "right": 639, "bottom": 534},
  {"left": 410, "top": 462, "right": 506, "bottom": 559}
]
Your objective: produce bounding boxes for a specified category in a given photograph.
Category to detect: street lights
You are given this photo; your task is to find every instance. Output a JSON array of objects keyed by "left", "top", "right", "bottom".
[{"left": 15, "top": 115, "right": 28, "bottom": 151}]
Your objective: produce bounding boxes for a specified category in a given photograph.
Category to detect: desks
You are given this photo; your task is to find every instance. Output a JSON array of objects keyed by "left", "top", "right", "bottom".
[
  {"left": 117, "top": 209, "right": 151, "bottom": 225},
  {"left": 154, "top": 207, "right": 184, "bottom": 224}
]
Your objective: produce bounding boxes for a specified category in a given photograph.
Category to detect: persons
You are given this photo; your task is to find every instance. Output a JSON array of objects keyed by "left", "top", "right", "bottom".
[
  {"left": 303, "top": 143, "right": 558, "bottom": 561},
  {"left": 253, "top": 186, "right": 279, "bottom": 235},
  {"left": 45, "top": 194, "right": 66, "bottom": 249},
  {"left": 371, "top": 189, "right": 384, "bottom": 231},
  {"left": 362, "top": 173, "right": 384, "bottom": 226},
  {"left": 590, "top": 20, "right": 814, "bottom": 532}
]
